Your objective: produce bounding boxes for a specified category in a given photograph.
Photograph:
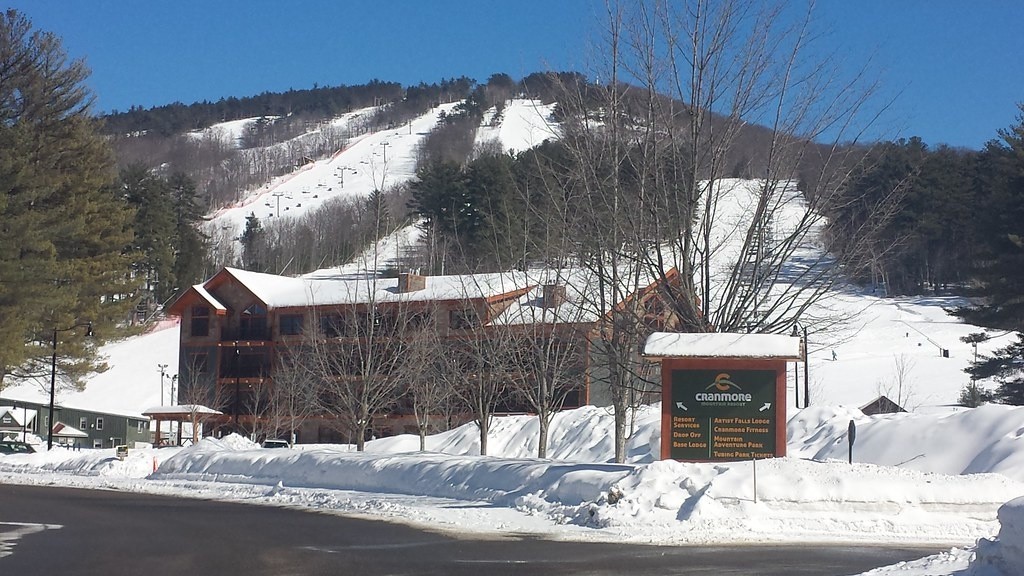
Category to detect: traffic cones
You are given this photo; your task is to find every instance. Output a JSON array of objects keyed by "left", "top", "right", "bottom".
[{"left": 153, "top": 457, "right": 159, "bottom": 473}]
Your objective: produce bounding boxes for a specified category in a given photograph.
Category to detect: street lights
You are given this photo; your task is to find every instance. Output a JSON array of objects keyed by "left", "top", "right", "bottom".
[
  {"left": 12, "top": 401, "right": 27, "bottom": 442},
  {"left": 158, "top": 364, "right": 169, "bottom": 406},
  {"left": 790, "top": 319, "right": 808, "bottom": 407},
  {"left": 233, "top": 339, "right": 242, "bottom": 432},
  {"left": 47, "top": 322, "right": 95, "bottom": 449},
  {"left": 166, "top": 373, "right": 178, "bottom": 432}
]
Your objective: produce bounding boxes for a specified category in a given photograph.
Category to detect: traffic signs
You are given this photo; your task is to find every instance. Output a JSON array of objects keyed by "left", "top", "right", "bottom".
[{"left": 660, "top": 359, "right": 787, "bottom": 463}]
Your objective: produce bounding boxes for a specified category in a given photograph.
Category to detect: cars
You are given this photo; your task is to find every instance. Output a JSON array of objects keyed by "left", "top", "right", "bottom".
[
  {"left": 261, "top": 438, "right": 289, "bottom": 449},
  {"left": 0, "top": 440, "right": 36, "bottom": 455}
]
